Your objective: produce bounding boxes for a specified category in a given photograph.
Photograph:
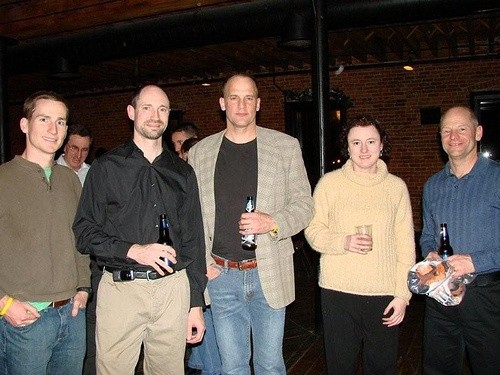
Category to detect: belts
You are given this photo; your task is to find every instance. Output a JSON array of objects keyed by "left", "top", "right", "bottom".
[
  {"left": 210, "top": 253, "right": 257, "bottom": 269},
  {"left": 49, "top": 298, "right": 71, "bottom": 307},
  {"left": 105, "top": 265, "right": 176, "bottom": 282}
]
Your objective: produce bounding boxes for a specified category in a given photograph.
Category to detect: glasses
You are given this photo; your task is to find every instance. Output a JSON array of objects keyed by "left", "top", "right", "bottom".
[{"left": 67, "top": 142, "right": 89, "bottom": 153}]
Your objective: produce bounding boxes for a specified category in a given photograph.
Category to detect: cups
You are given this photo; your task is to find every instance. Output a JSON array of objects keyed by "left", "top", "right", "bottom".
[{"left": 357, "top": 226, "right": 372, "bottom": 251}]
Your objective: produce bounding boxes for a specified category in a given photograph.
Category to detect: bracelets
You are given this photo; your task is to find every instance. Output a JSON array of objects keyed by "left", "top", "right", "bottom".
[
  {"left": 77, "top": 287, "right": 93, "bottom": 294},
  {"left": 274, "top": 223, "right": 278, "bottom": 234},
  {"left": 0, "top": 297, "right": 13, "bottom": 315}
]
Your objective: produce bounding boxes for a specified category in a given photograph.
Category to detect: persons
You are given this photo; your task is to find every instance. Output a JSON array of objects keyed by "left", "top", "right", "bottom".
[
  {"left": 0, "top": 91, "right": 91, "bottom": 375},
  {"left": 72, "top": 81, "right": 206, "bottom": 375},
  {"left": 56, "top": 124, "right": 91, "bottom": 186},
  {"left": 166, "top": 121, "right": 221, "bottom": 375},
  {"left": 187, "top": 72, "right": 316, "bottom": 375},
  {"left": 420, "top": 105, "right": 500, "bottom": 375},
  {"left": 303, "top": 114, "right": 416, "bottom": 374}
]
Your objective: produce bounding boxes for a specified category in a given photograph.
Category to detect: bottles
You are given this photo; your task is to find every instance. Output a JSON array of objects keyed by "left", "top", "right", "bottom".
[
  {"left": 437, "top": 222, "right": 453, "bottom": 268},
  {"left": 241, "top": 195, "right": 257, "bottom": 250},
  {"left": 157, "top": 213, "right": 175, "bottom": 274}
]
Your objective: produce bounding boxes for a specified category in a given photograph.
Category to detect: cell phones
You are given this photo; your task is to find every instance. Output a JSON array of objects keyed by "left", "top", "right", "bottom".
[{"left": 113, "top": 270, "right": 134, "bottom": 282}]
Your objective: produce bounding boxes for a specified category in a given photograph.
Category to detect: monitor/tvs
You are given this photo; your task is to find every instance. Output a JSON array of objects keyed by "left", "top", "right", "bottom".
[
  {"left": 471, "top": 90, "right": 500, "bottom": 163},
  {"left": 283, "top": 100, "right": 346, "bottom": 156}
]
